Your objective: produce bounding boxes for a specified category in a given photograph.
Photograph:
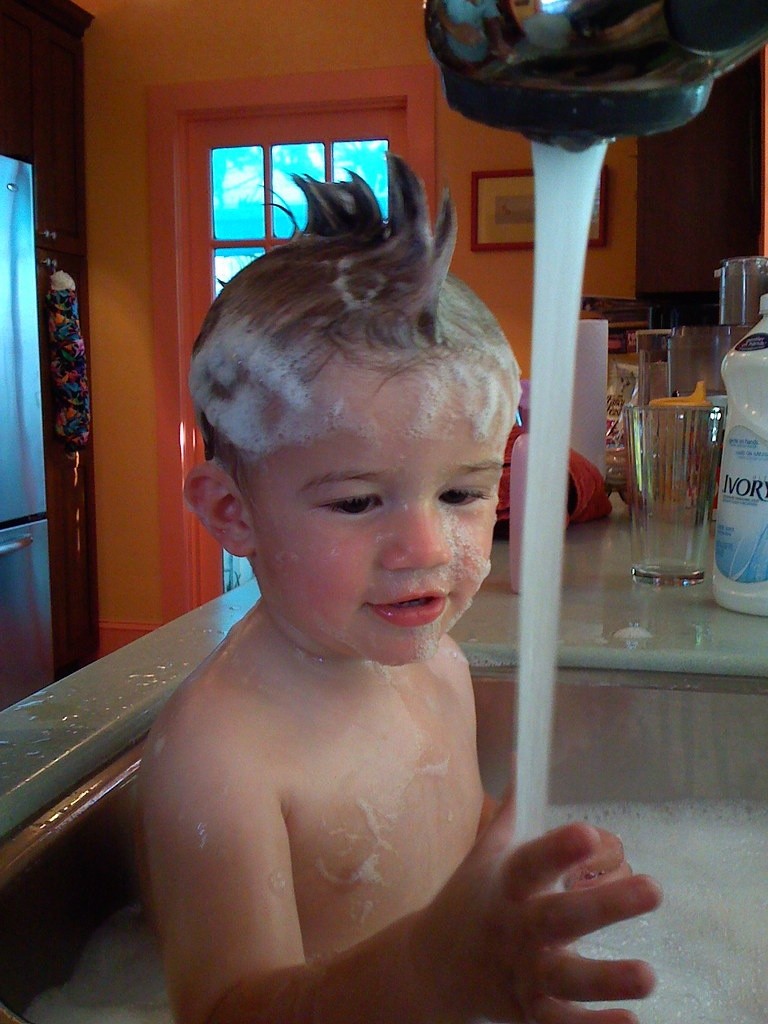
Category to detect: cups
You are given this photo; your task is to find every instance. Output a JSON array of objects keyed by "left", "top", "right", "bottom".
[
  {"left": 635, "top": 329, "right": 668, "bottom": 405},
  {"left": 719, "top": 256, "right": 768, "bottom": 326},
  {"left": 621, "top": 405, "right": 723, "bottom": 588},
  {"left": 668, "top": 328, "right": 731, "bottom": 398}
]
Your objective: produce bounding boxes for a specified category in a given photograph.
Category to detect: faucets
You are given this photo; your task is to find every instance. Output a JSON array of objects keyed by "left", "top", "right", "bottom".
[{"left": 419, "top": 0, "right": 768, "bottom": 152}]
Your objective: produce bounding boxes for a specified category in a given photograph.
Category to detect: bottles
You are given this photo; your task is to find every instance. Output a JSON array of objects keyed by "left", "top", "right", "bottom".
[{"left": 713, "top": 293, "right": 768, "bottom": 616}]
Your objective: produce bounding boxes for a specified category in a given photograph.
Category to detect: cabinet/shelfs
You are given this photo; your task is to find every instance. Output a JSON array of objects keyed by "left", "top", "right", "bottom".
[{"left": 0, "top": 0, "right": 104, "bottom": 684}]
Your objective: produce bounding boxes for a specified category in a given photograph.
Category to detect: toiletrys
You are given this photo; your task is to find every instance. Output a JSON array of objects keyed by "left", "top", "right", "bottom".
[{"left": 709, "top": 294, "right": 767, "bottom": 617}]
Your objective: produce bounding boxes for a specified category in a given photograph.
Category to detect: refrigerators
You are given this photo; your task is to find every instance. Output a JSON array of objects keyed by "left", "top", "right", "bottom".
[{"left": 0, "top": 156, "right": 56, "bottom": 714}]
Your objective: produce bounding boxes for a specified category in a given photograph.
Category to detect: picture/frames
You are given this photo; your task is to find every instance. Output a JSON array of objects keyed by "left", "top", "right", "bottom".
[{"left": 470, "top": 163, "right": 609, "bottom": 251}]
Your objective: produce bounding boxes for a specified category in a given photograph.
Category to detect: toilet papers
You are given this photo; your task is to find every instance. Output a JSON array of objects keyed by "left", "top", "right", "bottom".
[{"left": 569, "top": 318, "right": 606, "bottom": 492}]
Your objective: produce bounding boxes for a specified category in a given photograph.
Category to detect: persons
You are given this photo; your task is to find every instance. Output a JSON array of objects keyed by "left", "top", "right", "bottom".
[{"left": 136, "top": 150, "right": 663, "bottom": 1024}]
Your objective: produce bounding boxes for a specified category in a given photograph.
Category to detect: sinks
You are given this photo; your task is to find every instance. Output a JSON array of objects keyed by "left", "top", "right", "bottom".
[{"left": 0, "top": 633, "right": 768, "bottom": 1023}]
